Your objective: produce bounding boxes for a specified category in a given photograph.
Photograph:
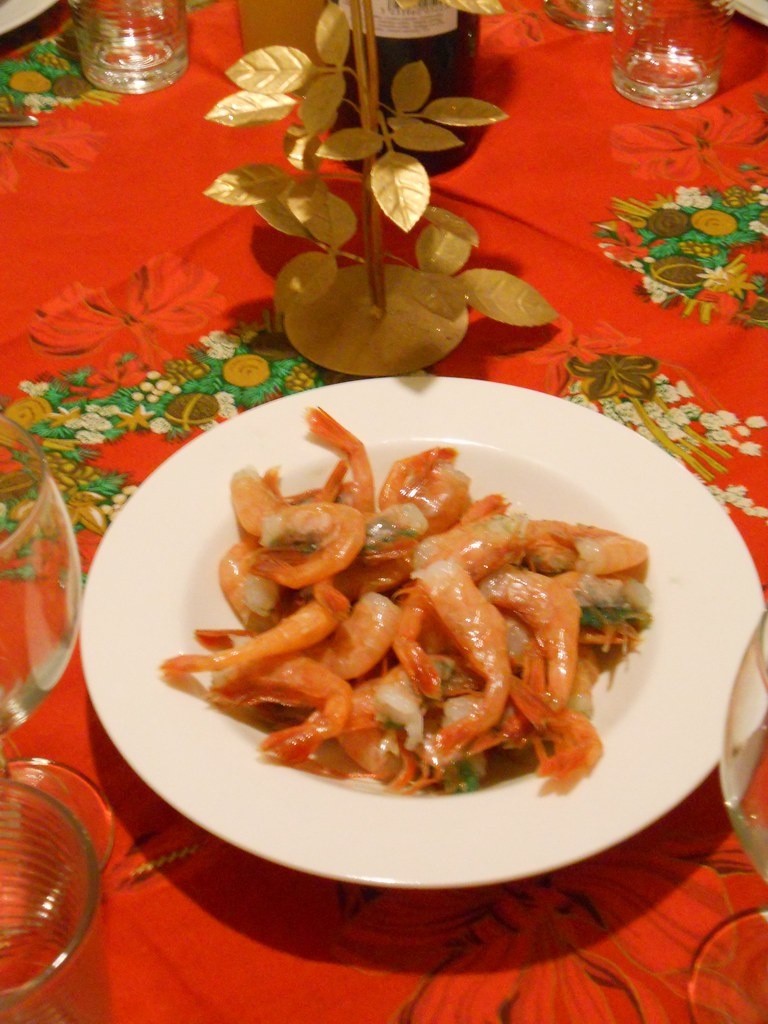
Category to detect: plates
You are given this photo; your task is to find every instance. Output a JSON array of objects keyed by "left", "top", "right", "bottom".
[
  {"left": 0, "top": 0, "right": 60, "bottom": 35},
  {"left": 75, "top": 375, "right": 768, "bottom": 891}
]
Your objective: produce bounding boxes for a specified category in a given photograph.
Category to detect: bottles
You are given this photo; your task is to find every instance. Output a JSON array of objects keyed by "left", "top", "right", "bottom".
[
  {"left": 326, "top": 1, "right": 481, "bottom": 177},
  {"left": 65, "top": 0, "right": 190, "bottom": 95}
]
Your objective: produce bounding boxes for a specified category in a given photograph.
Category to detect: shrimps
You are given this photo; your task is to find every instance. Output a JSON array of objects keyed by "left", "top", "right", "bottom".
[{"left": 162, "top": 409, "right": 650, "bottom": 793}]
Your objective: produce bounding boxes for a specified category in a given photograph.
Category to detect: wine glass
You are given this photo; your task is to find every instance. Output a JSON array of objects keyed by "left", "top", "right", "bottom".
[
  {"left": 0, "top": 413, "right": 116, "bottom": 880},
  {"left": 686, "top": 610, "right": 768, "bottom": 1024}
]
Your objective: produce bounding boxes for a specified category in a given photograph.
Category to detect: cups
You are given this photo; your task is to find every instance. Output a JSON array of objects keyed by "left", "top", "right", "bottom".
[
  {"left": 1, "top": 779, "right": 121, "bottom": 1024},
  {"left": 608, "top": 0, "right": 729, "bottom": 111},
  {"left": 541, "top": 0, "right": 616, "bottom": 33}
]
